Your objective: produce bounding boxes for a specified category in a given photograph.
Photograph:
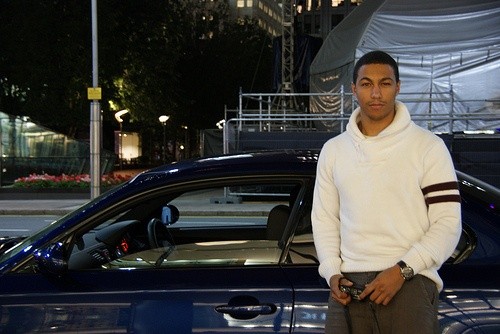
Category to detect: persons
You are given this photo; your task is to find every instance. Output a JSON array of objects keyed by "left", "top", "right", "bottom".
[{"left": 311, "top": 49, "right": 463, "bottom": 334}]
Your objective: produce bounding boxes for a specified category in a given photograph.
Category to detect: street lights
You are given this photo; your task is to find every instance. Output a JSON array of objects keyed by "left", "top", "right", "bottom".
[{"left": 158, "top": 113, "right": 169, "bottom": 167}]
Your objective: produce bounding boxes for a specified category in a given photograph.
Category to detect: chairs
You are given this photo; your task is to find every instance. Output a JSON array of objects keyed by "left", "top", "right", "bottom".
[{"left": 265, "top": 204, "right": 290, "bottom": 240}]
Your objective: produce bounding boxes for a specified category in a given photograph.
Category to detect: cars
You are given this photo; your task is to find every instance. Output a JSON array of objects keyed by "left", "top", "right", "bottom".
[{"left": 1, "top": 146, "right": 500, "bottom": 334}]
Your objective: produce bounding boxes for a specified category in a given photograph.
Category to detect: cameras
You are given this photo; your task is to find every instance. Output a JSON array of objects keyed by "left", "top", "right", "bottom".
[{"left": 338, "top": 285, "right": 366, "bottom": 302}]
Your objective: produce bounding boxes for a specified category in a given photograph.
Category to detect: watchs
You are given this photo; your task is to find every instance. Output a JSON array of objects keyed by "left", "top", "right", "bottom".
[{"left": 397, "top": 260, "right": 414, "bottom": 279}]
